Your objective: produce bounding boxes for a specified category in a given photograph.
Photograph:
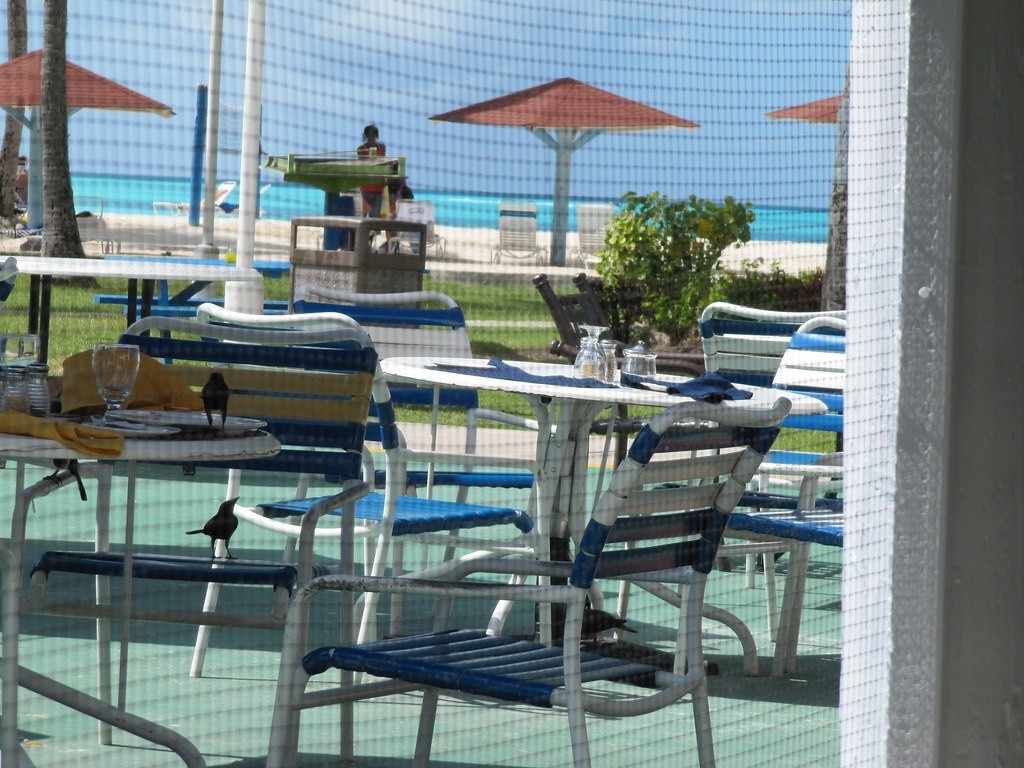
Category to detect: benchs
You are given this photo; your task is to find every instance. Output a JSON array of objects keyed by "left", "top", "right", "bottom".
[
  {"left": 124, "top": 305, "right": 288, "bottom": 317},
  {"left": 94, "top": 294, "right": 288, "bottom": 310}
]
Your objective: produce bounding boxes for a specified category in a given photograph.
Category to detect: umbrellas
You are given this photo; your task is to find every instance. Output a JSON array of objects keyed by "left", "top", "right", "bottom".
[
  {"left": 0, "top": 48, "right": 175, "bottom": 230},
  {"left": 428, "top": 77, "right": 701, "bottom": 266},
  {"left": 765, "top": 96, "right": 841, "bottom": 122}
]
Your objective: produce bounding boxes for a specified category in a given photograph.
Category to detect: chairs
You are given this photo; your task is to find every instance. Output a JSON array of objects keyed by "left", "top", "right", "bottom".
[
  {"left": 492, "top": 199, "right": 548, "bottom": 266},
  {"left": 29, "top": 285, "right": 846, "bottom": 768},
  {"left": 154, "top": 181, "right": 271, "bottom": 220},
  {"left": 389, "top": 198, "right": 446, "bottom": 258},
  {"left": 570, "top": 202, "right": 613, "bottom": 269},
  {"left": 76, "top": 216, "right": 121, "bottom": 254}
]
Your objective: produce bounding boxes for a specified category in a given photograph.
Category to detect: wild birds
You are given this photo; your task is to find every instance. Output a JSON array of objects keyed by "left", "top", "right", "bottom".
[
  {"left": 552, "top": 609, "right": 639, "bottom": 648},
  {"left": 201, "top": 372, "right": 231, "bottom": 432},
  {"left": 185, "top": 496, "right": 239, "bottom": 561}
]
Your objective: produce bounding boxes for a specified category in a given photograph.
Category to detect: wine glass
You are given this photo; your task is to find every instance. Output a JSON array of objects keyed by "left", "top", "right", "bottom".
[
  {"left": 0, "top": 334, "right": 40, "bottom": 371},
  {"left": 574, "top": 325, "right": 610, "bottom": 382},
  {"left": 92, "top": 343, "right": 140, "bottom": 426}
]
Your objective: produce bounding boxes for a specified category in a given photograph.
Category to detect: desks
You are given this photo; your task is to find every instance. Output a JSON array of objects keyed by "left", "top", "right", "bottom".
[
  {"left": 106, "top": 254, "right": 430, "bottom": 365},
  {"left": 0, "top": 256, "right": 262, "bottom": 364},
  {"left": 0, "top": 429, "right": 281, "bottom": 768},
  {"left": 380, "top": 356, "right": 828, "bottom": 676}
]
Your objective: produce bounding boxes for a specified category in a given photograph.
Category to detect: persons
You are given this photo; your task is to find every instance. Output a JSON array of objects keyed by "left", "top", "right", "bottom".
[
  {"left": 357, "top": 124, "right": 386, "bottom": 217},
  {"left": 12, "top": 156, "right": 27, "bottom": 208}
]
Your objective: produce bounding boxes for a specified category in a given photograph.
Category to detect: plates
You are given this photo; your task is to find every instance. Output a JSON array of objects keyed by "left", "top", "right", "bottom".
[
  {"left": 81, "top": 422, "right": 181, "bottom": 437},
  {"left": 105, "top": 410, "right": 267, "bottom": 429}
]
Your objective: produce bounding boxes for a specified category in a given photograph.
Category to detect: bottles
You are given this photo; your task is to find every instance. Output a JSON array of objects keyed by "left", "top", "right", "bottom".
[
  {"left": 599, "top": 339, "right": 619, "bottom": 381},
  {"left": 0, "top": 364, "right": 55, "bottom": 419}
]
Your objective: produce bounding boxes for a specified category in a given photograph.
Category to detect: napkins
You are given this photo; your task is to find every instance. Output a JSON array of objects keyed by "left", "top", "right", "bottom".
[
  {"left": 0, "top": 408, "right": 124, "bottom": 458},
  {"left": 62, "top": 347, "right": 202, "bottom": 414},
  {"left": 622, "top": 373, "right": 753, "bottom": 404},
  {"left": 434, "top": 359, "right": 620, "bottom": 388}
]
